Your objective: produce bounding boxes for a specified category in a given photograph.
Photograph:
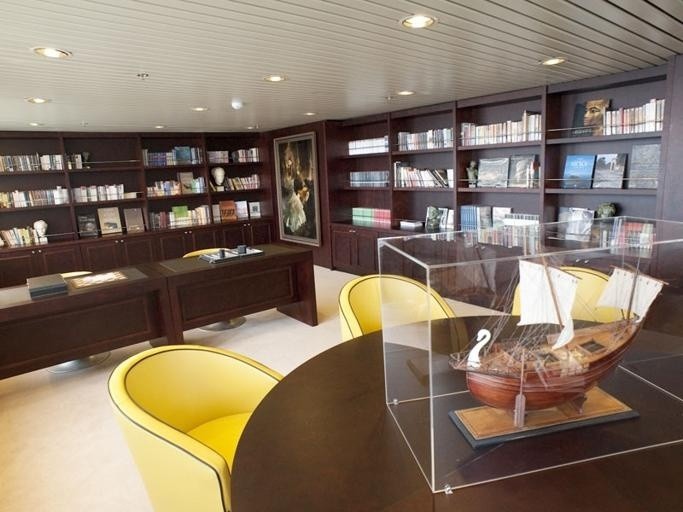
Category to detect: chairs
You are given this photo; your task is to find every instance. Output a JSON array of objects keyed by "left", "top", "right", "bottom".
[
  {"left": 108, "top": 342, "right": 284, "bottom": 512},
  {"left": 338, "top": 273, "right": 455, "bottom": 345}
]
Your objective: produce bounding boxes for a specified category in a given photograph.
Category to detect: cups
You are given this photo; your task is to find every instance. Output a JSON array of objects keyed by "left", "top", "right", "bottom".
[{"left": 219, "top": 250, "right": 225, "bottom": 258}]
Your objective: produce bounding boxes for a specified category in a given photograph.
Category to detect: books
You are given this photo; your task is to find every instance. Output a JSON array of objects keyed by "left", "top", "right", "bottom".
[
  {"left": 462, "top": 109, "right": 542, "bottom": 257},
  {"left": 0, "top": 143, "right": 262, "bottom": 249},
  {"left": 348, "top": 135, "right": 390, "bottom": 229},
  {"left": 393, "top": 128, "right": 453, "bottom": 241},
  {"left": 556, "top": 97, "right": 666, "bottom": 258}
]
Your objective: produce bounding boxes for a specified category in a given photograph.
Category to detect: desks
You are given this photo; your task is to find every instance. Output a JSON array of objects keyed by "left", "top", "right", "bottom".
[{"left": 228, "top": 312, "right": 683, "bottom": 512}]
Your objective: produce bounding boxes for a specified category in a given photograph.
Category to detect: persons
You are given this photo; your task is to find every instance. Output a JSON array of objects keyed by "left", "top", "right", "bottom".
[{"left": 280, "top": 145, "right": 309, "bottom": 236}]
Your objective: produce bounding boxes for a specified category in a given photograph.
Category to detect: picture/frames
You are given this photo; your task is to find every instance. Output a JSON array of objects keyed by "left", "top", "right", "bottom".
[{"left": 273, "top": 131, "right": 322, "bottom": 247}]
